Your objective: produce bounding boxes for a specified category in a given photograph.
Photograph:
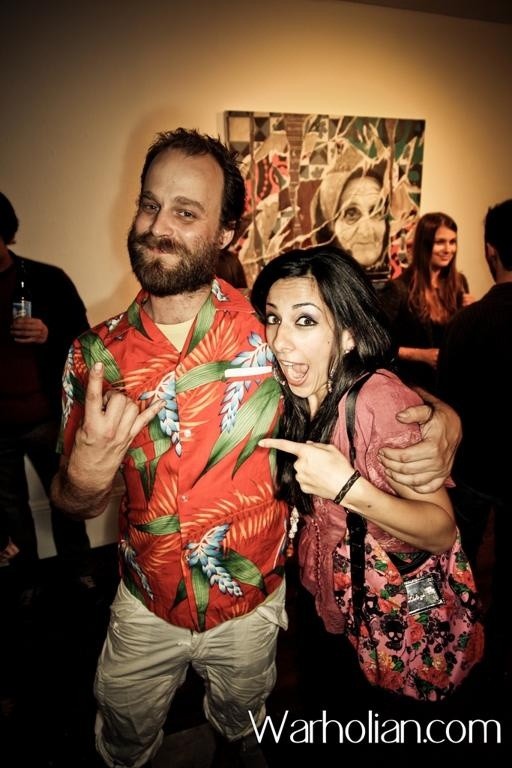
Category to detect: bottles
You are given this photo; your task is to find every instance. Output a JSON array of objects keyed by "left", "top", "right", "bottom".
[{"left": 8, "top": 258, "right": 33, "bottom": 341}]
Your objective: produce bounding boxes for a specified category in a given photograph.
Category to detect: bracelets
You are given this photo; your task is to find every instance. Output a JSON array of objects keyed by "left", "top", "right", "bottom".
[{"left": 332, "top": 470, "right": 360, "bottom": 506}]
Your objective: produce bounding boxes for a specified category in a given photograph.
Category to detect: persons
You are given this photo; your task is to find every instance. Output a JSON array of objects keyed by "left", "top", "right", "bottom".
[
  {"left": 429, "top": 196, "right": 511, "bottom": 680},
  {"left": 1, "top": 190, "right": 121, "bottom": 617},
  {"left": 1, "top": 528, "right": 22, "bottom": 563},
  {"left": 241, "top": 242, "right": 487, "bottom": 764},
  {"left": 48, "top": 123, "right": 468, "bottom": 766},
  {"left": 310, "top": 141, "right": 387, "bottom": 272},
  {"left": 374, "top": 211, "right": 477, "bottom": 399}
]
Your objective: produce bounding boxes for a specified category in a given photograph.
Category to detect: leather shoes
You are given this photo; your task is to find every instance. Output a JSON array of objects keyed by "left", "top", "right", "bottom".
[{"left": 14, "top": 575, "right": 102, "bottom": 607}]
